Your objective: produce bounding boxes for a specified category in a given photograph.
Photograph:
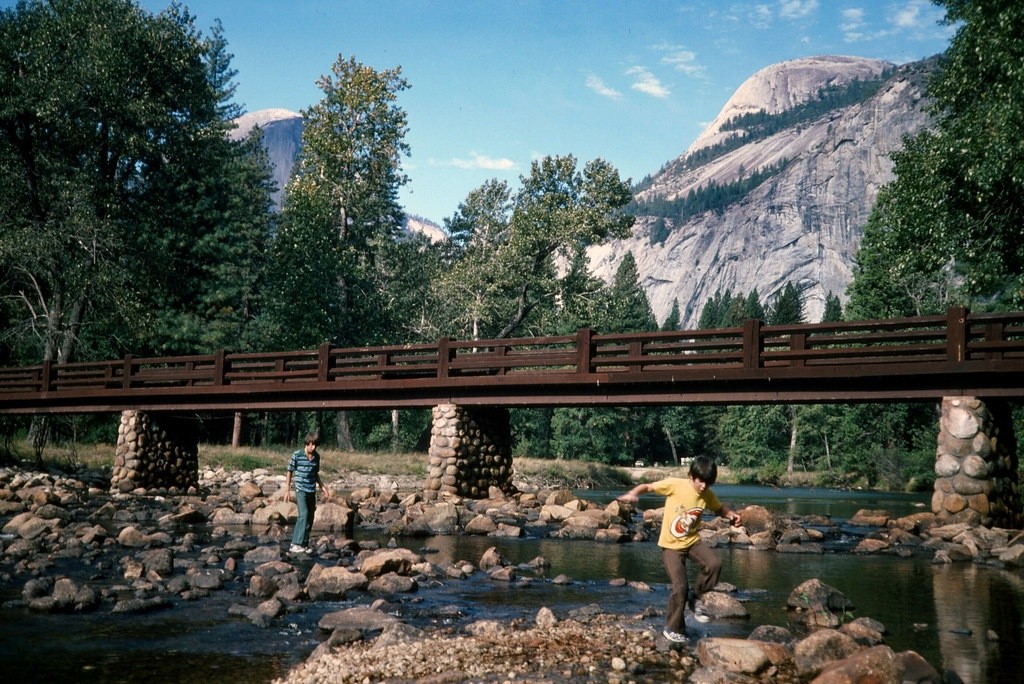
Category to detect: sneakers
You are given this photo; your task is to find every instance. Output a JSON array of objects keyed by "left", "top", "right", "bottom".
[
  {"left": 663, "top": 626, "right": 687, "bottom": 643},
  {"left": 303, "top": 545, "right": 313, "bottom": 554},
  {"left": 289, "top": 543, "right": 305, "bottom": 553},
  {"left": 688, "top": 596, "right": 711, "bottom": 624}
]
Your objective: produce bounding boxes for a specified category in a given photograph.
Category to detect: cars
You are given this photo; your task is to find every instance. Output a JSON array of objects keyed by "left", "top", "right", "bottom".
[{"left": 635, "top": 461, "right": 644, "bottom": 467}]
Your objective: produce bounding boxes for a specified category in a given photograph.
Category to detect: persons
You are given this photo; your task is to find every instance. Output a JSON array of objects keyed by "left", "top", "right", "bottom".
[
  {"left": 616, "top": 457, "right": 743, "bottom": 643},
  {"left": 284, "top": 430, "right": 331, "bottom": 554}
]
